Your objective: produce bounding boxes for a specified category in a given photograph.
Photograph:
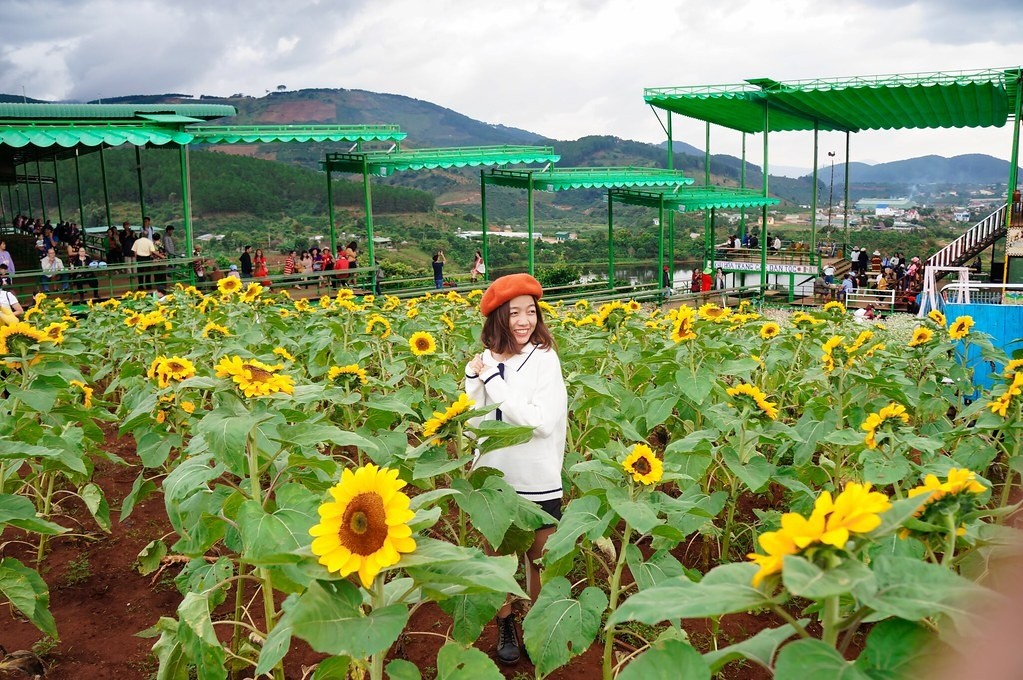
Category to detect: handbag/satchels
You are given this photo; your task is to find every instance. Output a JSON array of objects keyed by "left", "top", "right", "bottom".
[{"left": 478, "top": 263, "right": 486, "bottom": 274}]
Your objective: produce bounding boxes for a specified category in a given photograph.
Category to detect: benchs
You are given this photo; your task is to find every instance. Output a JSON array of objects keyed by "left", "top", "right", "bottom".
[
  {"left": 88, "top": 234, "right": 106, "bottom": 248},
  {"left": 87, "top": 244, "right": 106, "bottom": 260}
]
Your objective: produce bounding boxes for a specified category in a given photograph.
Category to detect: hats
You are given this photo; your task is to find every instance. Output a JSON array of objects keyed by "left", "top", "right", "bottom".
[
  {"left": 309, "top": 245, "right": 321, "bottom": 253},
  {"left": 123, "top": 222, "right": 129, "bottom": 226},
  {"left": 872, "top": 251, "right": 881, "bottom": 255},
  {"left": 912, "top": 257, "right": 919, "bottom": 261},
  {"left": 322, "top": 246, "right": 331, "bottom": 252},
  {"left": 663, "top": 266, "right": 669, "bottom": 270},
  {"left": 702, "top": 268, "right": 712, "bottom": 274},
  {"left": 861, "top": 248, "right": 866, "bottom": 251},
  {"left": 479, "top": 272, "right": 542, "bottom": 318},
  {"left": 194, "top": 244, "right": 202, "bottom": 249},
  {"left": 907, "top": 296, "right": 915, "bottom": 301},
  {"left": 848, "top": 270, "right": 856, "bottom": 276},
  {"left": 900, "top": 264, "right": 904, "bottom": 267},
  {"left": 229, "top": 264, "right": 237, "bottom": 271},
  {"left": 853, "top": 246, "right": 859, "bottom": 251}
]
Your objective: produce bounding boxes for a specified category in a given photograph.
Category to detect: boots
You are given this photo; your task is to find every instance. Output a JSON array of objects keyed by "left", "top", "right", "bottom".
[{"left": 494, "top": 613, "right": 521, "bottom": 666}]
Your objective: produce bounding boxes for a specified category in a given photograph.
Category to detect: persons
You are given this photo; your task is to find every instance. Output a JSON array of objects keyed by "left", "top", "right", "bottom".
[
  {"left": 431, "top": 249, "right": 447, "bottom": 290},
  {"left": 662, "top": 265, "right": 672, "bottom": 297},
  {"left": 470, "top": 251, "right": 485, "bottom": 283},
  {"left": 688, "top": 231, "right": 922, "bottom": 321},
  {"left": 0, "top": 215, "right": 386, "bottom": 299},
  {"left": 0, "top": 289, "right": 26, "bottom": 400},
  {"left": 460, "top": 272, "right": 572, "bottom": 666}
]
